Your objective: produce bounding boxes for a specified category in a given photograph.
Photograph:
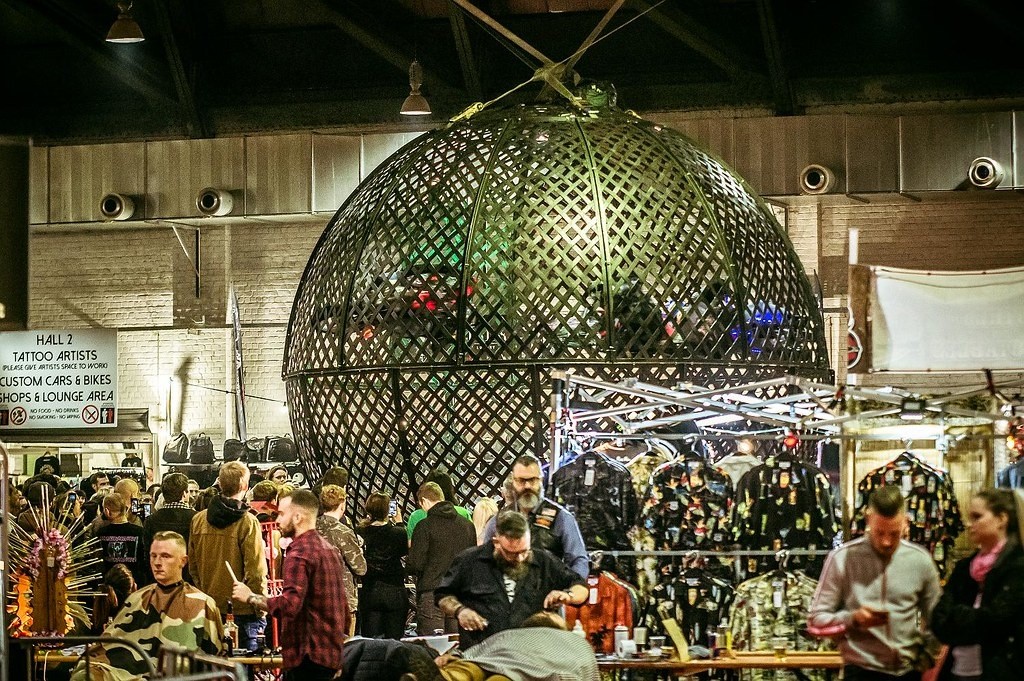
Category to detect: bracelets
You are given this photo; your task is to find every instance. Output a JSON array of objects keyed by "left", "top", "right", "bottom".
[
  {"left": 454, "top": 605, "right": 468, "bottom": 617},
  {"left": 563, "top": 589, "right": 572, "bottom": 604}
]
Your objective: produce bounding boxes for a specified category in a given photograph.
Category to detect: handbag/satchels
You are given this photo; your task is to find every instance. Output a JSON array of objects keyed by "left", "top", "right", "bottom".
[{"left": 164, "top": 431, "right": 297, "bottom": 463}]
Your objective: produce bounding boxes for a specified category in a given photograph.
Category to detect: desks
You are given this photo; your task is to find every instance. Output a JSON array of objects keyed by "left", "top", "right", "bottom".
[{"left": 34, "top": 647, "right": 284, "bottom": 681}]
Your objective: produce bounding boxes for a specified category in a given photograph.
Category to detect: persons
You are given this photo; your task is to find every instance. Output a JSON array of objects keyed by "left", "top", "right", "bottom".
[
  {"left": 398, "top": 611, "right": 598, "bottom": 681},
  {"left": 233, "top": 490, "right": 349, "bottom": 681},
  {"left": 316, "top": 483, "right": 366, "bottom": 637},
  {"left": 80, "top": 531, "right": 227, "bottom": 681},
  {"left": 91, "top": 561, "right": 138, "bottom": 632},
  {"left": 480, "top": 456, "right": 590, "bottom": 581},
  {"left": 930, "top": 488, "right": 1024, "bottom": 680},
  {"left": 808, "top": 483, "right": 944, "bottom": 681},
  {"left": 6, "top": 464, "right": 516, "bottom": 595},
  {"left": 186, "top": 462, "right": 270, "bottom": 654},
  {"left": 409, "top": 480, "right": 477, "bottom": 636},
  {"left": 355, "top": 490, "right": 409, "bottom": 639},
  {"left": 434, "top": 509, "right": 589, "bottom": 652}
]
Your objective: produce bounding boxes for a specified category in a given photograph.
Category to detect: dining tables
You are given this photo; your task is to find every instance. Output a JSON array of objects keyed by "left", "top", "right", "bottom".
[{"left": 596, "top": 649, "right": 844, "bottom": 681}]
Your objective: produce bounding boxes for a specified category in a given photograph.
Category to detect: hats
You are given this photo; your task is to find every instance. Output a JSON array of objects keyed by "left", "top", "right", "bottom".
[
  {"left": 28, "top": 482, "right": 54, "bottom": 505},
  {"left": 268, "top": 466, "right": 287, "bottom": 481},
  {"left": 53, "top": 482, "right": 78, "bottom": 497}
]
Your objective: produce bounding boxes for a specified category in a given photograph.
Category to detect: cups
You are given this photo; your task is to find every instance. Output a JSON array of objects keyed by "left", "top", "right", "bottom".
[
  {"left": 650, "top": 636, "right": 665, "bottom": 649},
  {"left": 773, "top": 637, "right": 787, "bottom": 658}
]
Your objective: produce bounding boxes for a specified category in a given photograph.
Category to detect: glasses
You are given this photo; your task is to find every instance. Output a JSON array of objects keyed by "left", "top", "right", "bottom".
[{"left": 513, "top": 477, "right": 539, "bottom": 486}]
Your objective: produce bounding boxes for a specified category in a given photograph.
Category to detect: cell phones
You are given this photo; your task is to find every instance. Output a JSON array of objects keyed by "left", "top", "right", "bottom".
[{"left": 872, "top": 608, "right": 889, "bottom": 624}]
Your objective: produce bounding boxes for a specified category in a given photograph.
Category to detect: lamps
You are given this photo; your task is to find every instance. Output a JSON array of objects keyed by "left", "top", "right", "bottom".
[
  {"left": 397, "top": 0, "right": 432, "bottom": 116},
  {"left": 105, "top": 0, "right": 145, "bottom": 43}
]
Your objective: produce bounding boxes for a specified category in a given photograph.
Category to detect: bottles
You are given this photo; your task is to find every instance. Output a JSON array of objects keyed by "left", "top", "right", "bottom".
[
  {"left": 716, "top": 617, "right": 732, "bottom": 653},
  {"left": 224, "top": 600, "right": 238, "bottom": 649},
  {"left": 107, "top": 617, "right": 113, "bottom": 627},
  {"left": 572, "top": 620, "right": 586, "bottom": 638}
]
